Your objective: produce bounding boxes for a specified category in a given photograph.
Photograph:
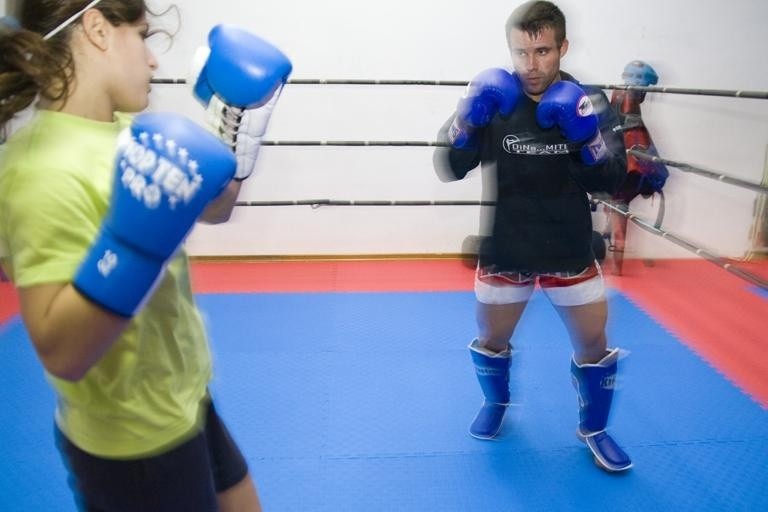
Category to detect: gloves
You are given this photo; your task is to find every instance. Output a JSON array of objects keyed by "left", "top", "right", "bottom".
[
  {"left": 448, "top": 67, "right": 521, "bottom": 151},
  {"left": 534, "top": 80, "right": 607, "bottom": 166},
  {"left": 72, "top": 108, "right": 237, "bottom": 319},
  {"left": 192, "top": 25, "right": 292, "bottom": 181}
]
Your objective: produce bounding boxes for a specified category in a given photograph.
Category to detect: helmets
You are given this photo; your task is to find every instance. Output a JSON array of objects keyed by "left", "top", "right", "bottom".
[{"left": 622, "top": 60, "right": 658, "bottom": 86}]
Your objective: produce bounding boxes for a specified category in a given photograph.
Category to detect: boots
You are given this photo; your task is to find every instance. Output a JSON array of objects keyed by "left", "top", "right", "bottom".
[
  {"left": 570, "top": 348, "right": 632, "bottom": 474},
  {"left": 466, "top": 337, "right": 510, "bottom": 440}
]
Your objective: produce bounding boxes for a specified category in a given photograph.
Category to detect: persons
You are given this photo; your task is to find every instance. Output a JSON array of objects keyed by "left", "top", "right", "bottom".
[
  {"left": 0, "top": 0, "right": 294, "bottom": 511},
  {"left": 428, "top": 0, "right": 636, "bottom": 479},
  {"left": 600, "top": 58, "right": 670, "bottom": 278}
]
